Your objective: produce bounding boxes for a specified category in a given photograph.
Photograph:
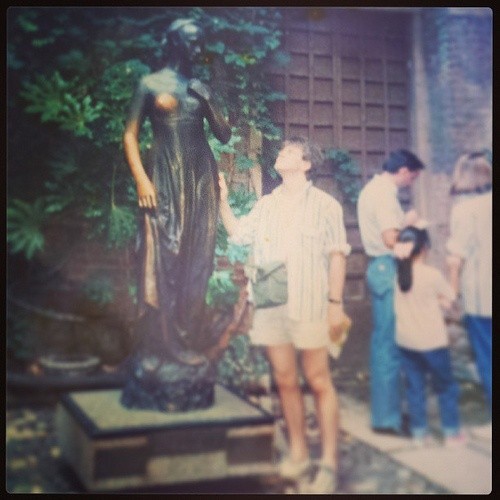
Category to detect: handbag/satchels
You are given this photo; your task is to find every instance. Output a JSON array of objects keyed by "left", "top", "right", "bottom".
[{"left": 251, "top": 262, "right": 290, "bottom": 307}]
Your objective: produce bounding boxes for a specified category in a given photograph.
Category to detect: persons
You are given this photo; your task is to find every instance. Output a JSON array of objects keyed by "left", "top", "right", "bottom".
[
  {"left": 122, "top": 16, "right": 233, "bottom": 405},
  {"left": 213, "top": 133, "right": 354, "bottom": 492},
  {"left": 386, "top": 226, "right": 465, "bottom": 442},
  {"left": 447, "top": 153, "right": 493, "bottom": 418},
  {"left": 355, "top": 149, "right": 424, "bottom": 435}
]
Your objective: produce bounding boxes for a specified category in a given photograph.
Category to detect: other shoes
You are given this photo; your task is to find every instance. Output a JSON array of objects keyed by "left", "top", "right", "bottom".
[
  {"left": 374, "top": 417, "right": 415, "bottom": 439},
  {"left": 306, "top": 459, "right": 340, "bottom": 494},
  {"left": 280, "top": 454, "right": 311, "bottom": 477}
]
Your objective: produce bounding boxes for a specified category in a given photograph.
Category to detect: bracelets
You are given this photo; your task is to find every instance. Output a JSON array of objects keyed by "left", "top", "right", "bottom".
[{"left": 330, "top": 297, "right": 342, "bottom": 305}]
